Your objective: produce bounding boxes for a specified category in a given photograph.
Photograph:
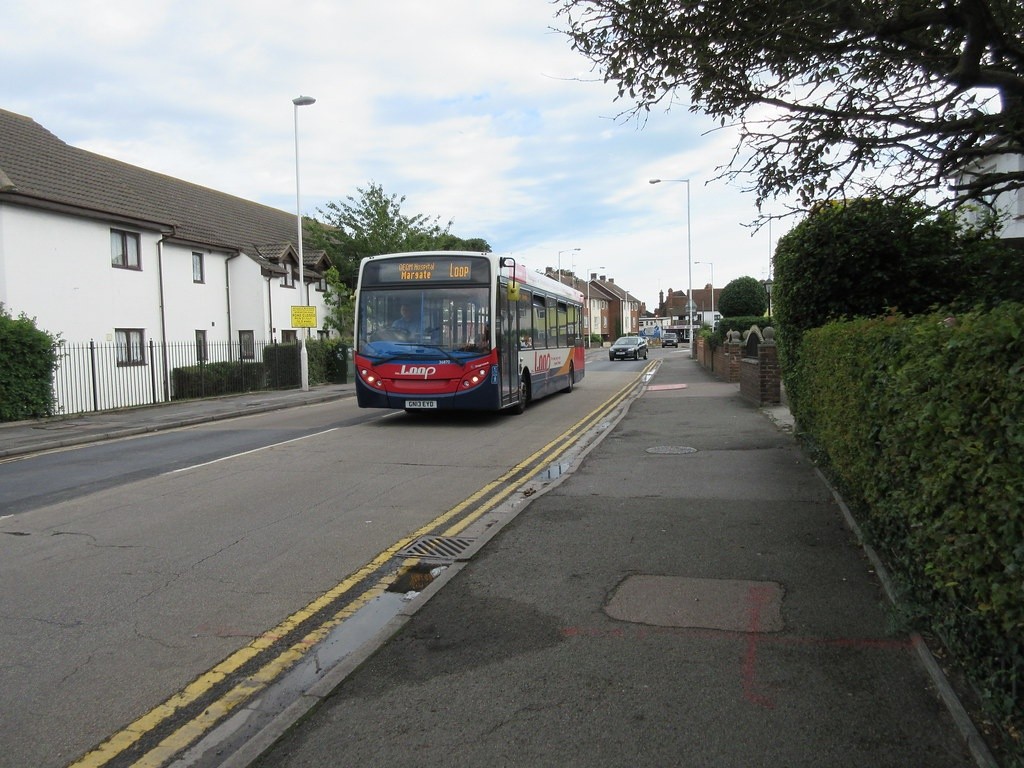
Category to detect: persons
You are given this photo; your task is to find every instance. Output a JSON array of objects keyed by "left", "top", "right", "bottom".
[{"left": 392, "top": 304, "right": 421, "bottom": 333}]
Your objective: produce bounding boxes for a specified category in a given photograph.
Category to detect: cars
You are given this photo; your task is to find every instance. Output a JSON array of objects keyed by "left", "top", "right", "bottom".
[{"left": 610, "top": 336, "right": 649, "bottom": 361}]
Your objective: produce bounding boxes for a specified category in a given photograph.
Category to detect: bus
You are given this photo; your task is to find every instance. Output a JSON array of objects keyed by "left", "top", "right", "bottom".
[{"left": 349, "top": 250, "right": 586, "bottom": 416}]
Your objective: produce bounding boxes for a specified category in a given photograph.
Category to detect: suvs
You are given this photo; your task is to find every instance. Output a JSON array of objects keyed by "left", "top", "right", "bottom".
[{"left": 660, "top": 332, "right": 680, "bottom": 348}]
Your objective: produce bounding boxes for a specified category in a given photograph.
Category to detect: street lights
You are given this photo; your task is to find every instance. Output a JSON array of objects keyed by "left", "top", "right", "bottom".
[
  {"left": 586, "top": 266, "right": 606, "bottom": 348},
  {"left": 762, "top": 278, "right": 775, "bottom": 324},
  {"left": 757, "top": 213, "right": 772, "bottom": 277},
  {"left": 694, "top": 261, "right": 715, "bottom": 333},
  {"left": 292, "top": 95, "right": 317, "bottom": 392},
  {"left": 650, "top": 178, "right": 693, "bottom": 360},
  {"left": 558, "top": 248, "right": 581, "bottom": 284},
  {"left": 624, "top": 290, "right": 631, "bottom": 337}
]
